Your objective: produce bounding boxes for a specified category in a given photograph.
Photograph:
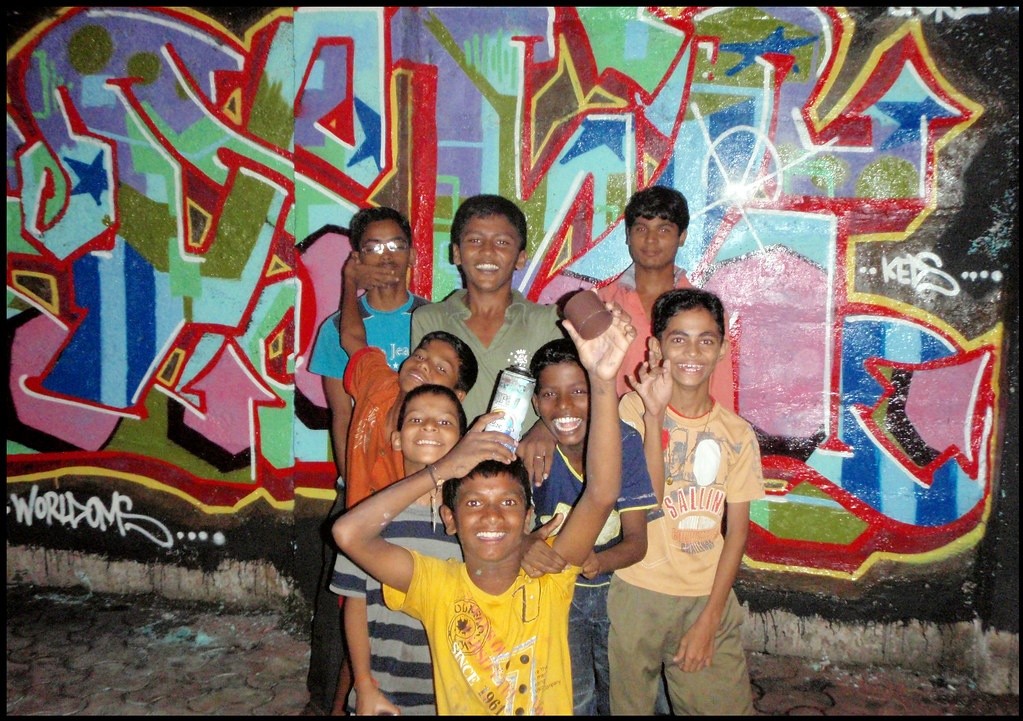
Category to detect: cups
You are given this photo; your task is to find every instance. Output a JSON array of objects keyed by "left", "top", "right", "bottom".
[{"left": 563, "top": 291, "right": 611, "bottom": 341}]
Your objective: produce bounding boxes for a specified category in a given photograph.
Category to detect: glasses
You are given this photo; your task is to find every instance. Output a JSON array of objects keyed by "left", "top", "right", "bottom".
[{"left": 359, "top": 239, "right": 408, "bottom": 254}]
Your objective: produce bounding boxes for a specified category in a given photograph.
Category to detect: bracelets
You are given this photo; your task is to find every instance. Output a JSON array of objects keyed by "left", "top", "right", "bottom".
[{"left": 425, "top": 463, "right": 438, "bottom": 491}]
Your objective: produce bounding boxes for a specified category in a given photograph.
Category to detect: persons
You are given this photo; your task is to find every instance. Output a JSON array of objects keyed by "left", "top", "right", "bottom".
[
  {"left": 598, "top": 185, "right": 734, "bottom": 419},
  {"left": 521, "top": 339, "right": 648, "bottom": 717},
  {"left": 315, "top": 252, "right": 479, "bottom": 716},
  {"left": 410, "top": 195, "right": 589, "bottom": 491},
  {"left": 298, "top": 208, "right": 434, "bottom": 717},
  {"left": 332, "top": 383, "right": 468, "bottom": 716},
  {"left": 606, "top": 289, "right": 765, "bottom": 715},
  {"left": 331, "top": 285, "right": 639, "bottom": 716}
]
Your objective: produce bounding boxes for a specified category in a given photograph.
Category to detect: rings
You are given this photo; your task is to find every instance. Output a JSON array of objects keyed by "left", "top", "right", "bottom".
[{"left": 533, "top": 455, "right": 544, "bottom": 460}]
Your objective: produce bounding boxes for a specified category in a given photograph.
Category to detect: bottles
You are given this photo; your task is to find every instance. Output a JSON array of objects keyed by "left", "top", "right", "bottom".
[{"left": 484, "top": 364, "right": 537, "bottom": 459}]
